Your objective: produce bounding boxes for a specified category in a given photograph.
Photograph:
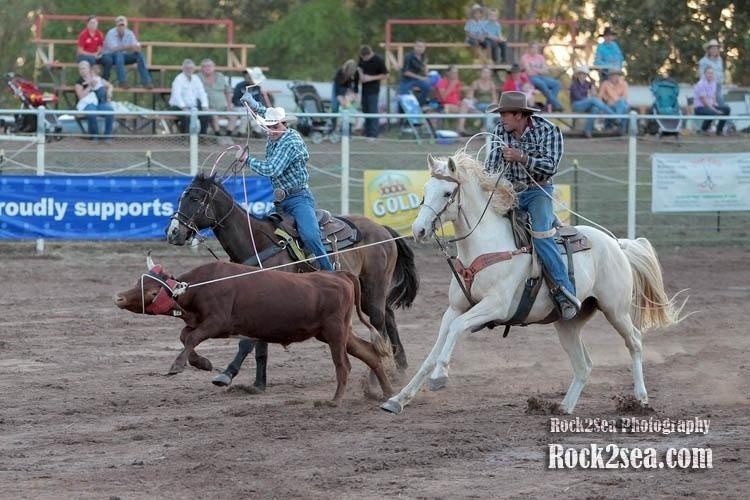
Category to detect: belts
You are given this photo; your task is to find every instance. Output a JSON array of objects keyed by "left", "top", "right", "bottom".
[
  {"left": 273, "top": 183, "right": 309, "bottom": 201},
  {"left": 511, "top": 180, "right": 551, "bottom": 194}
]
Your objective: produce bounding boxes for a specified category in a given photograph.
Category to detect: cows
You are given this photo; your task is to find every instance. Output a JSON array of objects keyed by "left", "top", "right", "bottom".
[{"left": 114, "top": 248, "right": 393, "bottom": 408}]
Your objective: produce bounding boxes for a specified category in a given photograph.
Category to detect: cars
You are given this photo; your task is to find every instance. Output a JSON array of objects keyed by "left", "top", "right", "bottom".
[{"left": 57, "top": 99, "right": 158, "bottom": 136}]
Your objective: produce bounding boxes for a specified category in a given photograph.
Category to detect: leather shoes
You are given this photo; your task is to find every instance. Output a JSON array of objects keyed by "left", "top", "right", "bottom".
[
  {"left": 717, "top": 132, "right": 727, "bottom": 137},
  {"left": 696, "top": 130, "right": 710, "bottom": 136}
]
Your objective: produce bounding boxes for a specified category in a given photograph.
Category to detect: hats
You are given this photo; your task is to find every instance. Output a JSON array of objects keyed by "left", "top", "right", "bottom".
[
  {"left": 256, "top": 107, "right": 298, "bottom": 126},
  {"left": 508, "top": 63, "right": 526, "bottom": 72},
  {"left": 470, "top": 4, "right": 484, "bottom": 12},
  {"left": 599, "top": 27, "right": 618, "bottom": 37},
  {"left": 608, "top": 67, "right": 624, "bottom": 75},
  {"left": 703, "top": 39, "right": 720, "bottom": 51},
  {"left": 485, "top": 91, "right": 541, "bottom": 113}
]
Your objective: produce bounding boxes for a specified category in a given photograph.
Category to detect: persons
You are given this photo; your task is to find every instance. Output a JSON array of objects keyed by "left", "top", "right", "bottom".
[
  {"left": 436, "top": 65, "right": 473, "bottom": 137},
  {"left": 700, "top": 40, "right": 723, "bottom": 103},
  {"left": 234, "top": 93, "right": 333, "bottom": 271},
  {"left": 484, "top": 89, "right": 578, "bottom": 323},
  {"left": 592, "top": 27, "right": 624, "bottom": 81},
  {"left": 518, "top": 42, "right": 568, "bottom": 112},
  {"left": 231, "top": 72, "right": 266, "bottom": 137},
  {"left": 397, "top": 39, "right": 430, "bottom": 128},
  {"left": 357, "top": 47, "right": 391, "bottom": 139},
  {"left": 482, "top": 7, "right": 510, "bottom": 65},
  {"left": 464, "top": 5, "right": 494, "bottom": 65},
  {"left": 692, "top": 65, "right": 731, "bottom": 136},
  {"left": 571, "top": 66, "right": 622, "bottom": 138},
  {"left": 106, "top": 16, "right": 155, "bottom": 91},
  {"left": 337, "top": 90, "right": 362, "bottom": 135},
  {"left": 58, "top": 64, "right": 113, "bottom": 122},
  {"left": 78, "top": 16, "right": 105, "bottom": 80},
  {"left": 503, "top": 64, "right": 553, "bottom": 113},
  {"left": 467, "top": 67, "right": 500, "bottom": 132},
  {"left": 194, "top": 61, "right": 236, "bottom": 136},
  {"left": 331, "top": 60, "right": 361, "bottom": 133},
  {"left": 597, "top": 69, "right": 628, "bottom": 134},
  {"left": 169, "top": 59, "right": 225, "bottom": 138},
  {"left": 76, "top": 61, "right": 116, "bottom": 142}
]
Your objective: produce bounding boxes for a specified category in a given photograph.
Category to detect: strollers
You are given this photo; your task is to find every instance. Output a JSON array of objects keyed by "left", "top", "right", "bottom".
[
  {"left": 647, "top": 77, "right": 682, "bottom": 141},
  {"left": 286, "top": 81, "right": 340, "bottom": 144},
  {"left": 4, "top": 72, "right": 63, "bottom": 142}
]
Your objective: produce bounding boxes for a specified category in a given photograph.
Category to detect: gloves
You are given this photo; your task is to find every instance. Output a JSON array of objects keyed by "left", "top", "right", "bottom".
[{"left": 240, "top": 92, "right": 259, "bottom": 111}]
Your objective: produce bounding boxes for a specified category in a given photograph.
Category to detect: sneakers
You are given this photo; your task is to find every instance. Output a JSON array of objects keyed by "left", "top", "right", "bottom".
[{"left": 561, "top": 299, "right": 577, "bottom": 320}]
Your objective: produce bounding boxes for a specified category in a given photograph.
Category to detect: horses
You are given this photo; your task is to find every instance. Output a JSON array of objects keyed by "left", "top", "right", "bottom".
[
  {"left": 380, "top": 145, "right": 700, "bottom": 415},
  {"left": 167, "top": 173, "right": 421, "bottom": 401}
]
